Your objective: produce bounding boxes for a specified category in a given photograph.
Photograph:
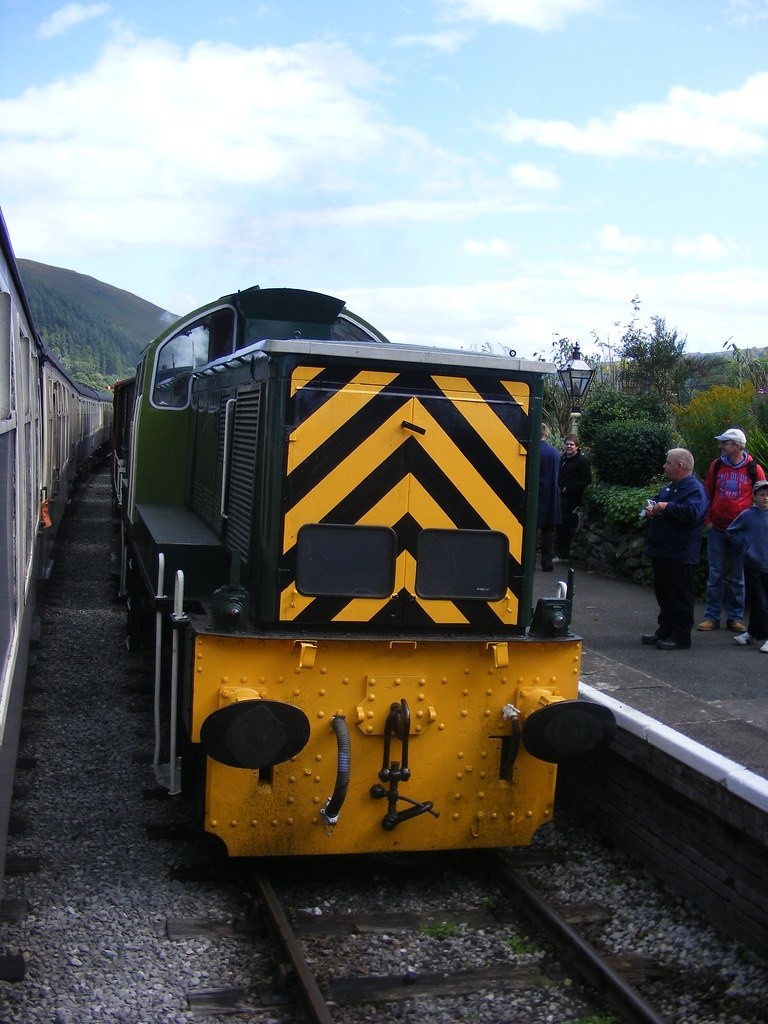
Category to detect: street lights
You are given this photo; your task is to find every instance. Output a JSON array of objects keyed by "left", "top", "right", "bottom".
[{"left": 556, "top": 340, "right": 599, "bottom": 442}]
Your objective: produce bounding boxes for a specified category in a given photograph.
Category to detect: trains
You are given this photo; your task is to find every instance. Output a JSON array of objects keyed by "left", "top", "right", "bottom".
[
  {"left": 105, "top": 285, "right": 615, "bottom": 883},
  {"left": 0, "top": 207, "right": 115, "bottom": 887}
]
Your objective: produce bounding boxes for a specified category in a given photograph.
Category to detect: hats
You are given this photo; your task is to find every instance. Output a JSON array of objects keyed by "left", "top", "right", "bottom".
[
  {"left": 714, "top": 428, "right": 747, "bottom": 445},
  {"left": 752, "top": 480, "right": 768, "bottom": 492}
]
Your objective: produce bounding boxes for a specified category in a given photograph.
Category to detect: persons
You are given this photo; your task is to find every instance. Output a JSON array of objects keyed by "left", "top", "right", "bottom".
[
  {"left": 534, "top": 422, "right": 592, "bottom": 572},
  {"left": 705, "top": 429, "right": 768, "bottom": 653},
  {"left": 641, "top": 449, "right": 709, "bottom": 651}
]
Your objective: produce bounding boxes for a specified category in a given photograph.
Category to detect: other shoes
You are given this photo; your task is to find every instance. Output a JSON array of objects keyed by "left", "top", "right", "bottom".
[
  {"left": 657, "top": 638, "right": 686, "bottom": 650},
  {"left": 551, "top": 557, "right": 568, "bottom": 563},
  {"left": 641, "top": 634, "right": 658, "bottom": 643},
  {"left": 542, "top": 566, "right": 554, "bottom": 572},
  {"left": 697, "top": 620, "right": 721, "bottom": 630},
  {"left": 760, "top": 640, "right": 768, "bottom": 651},
  {"left": 726, "top": 619, "right": 747, "bottom": 631},
  {"left": 733, "top": 631, "right": 753, "bottom": 644}
]
